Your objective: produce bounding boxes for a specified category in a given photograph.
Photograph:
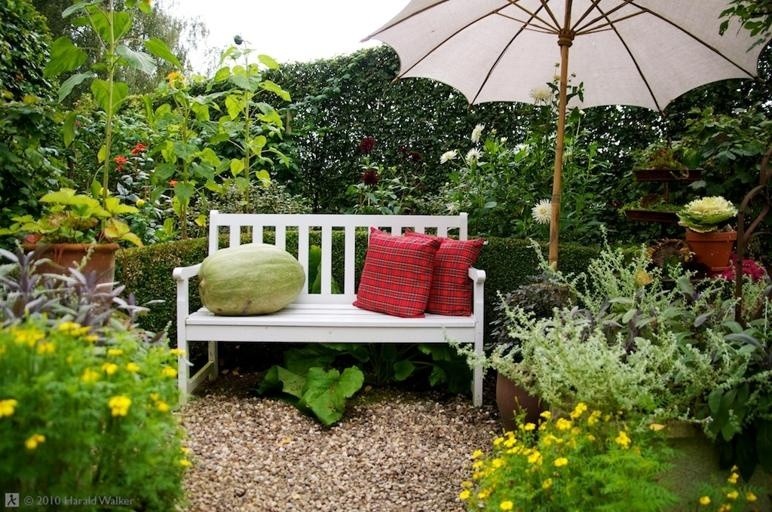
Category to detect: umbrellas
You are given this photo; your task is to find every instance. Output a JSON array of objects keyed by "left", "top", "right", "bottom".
[{"left": 359, "top": 1, "right": 771, "bottom": 280}]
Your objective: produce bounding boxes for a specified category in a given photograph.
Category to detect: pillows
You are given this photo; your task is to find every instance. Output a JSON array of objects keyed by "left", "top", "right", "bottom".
[{"left": 352, "top": 227, "right": 486, "bottom": 320}]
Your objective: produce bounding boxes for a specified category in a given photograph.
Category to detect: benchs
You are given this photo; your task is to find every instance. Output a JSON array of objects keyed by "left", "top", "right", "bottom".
[{"left": 172, "top": 208, "right": 486, "bottom": 412}]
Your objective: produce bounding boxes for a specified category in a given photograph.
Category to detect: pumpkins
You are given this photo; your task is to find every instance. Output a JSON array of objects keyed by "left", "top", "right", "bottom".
[{"left": 196, "top": 244, "right": 306, "bottom": 316}]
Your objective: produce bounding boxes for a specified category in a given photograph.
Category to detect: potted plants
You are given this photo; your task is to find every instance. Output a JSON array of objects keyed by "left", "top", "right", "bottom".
[
  {"left": 617, "top": 140, "right": 703, "bottom": 225},
  {"left": 679, "top": 196, "right": 740, "bottom": 272},
  {"left": 493, "top": 275, "right": 580, "bottom": 433},
  {"left": 0, "top": 189, "right": 142, "bottom": 326}
]
